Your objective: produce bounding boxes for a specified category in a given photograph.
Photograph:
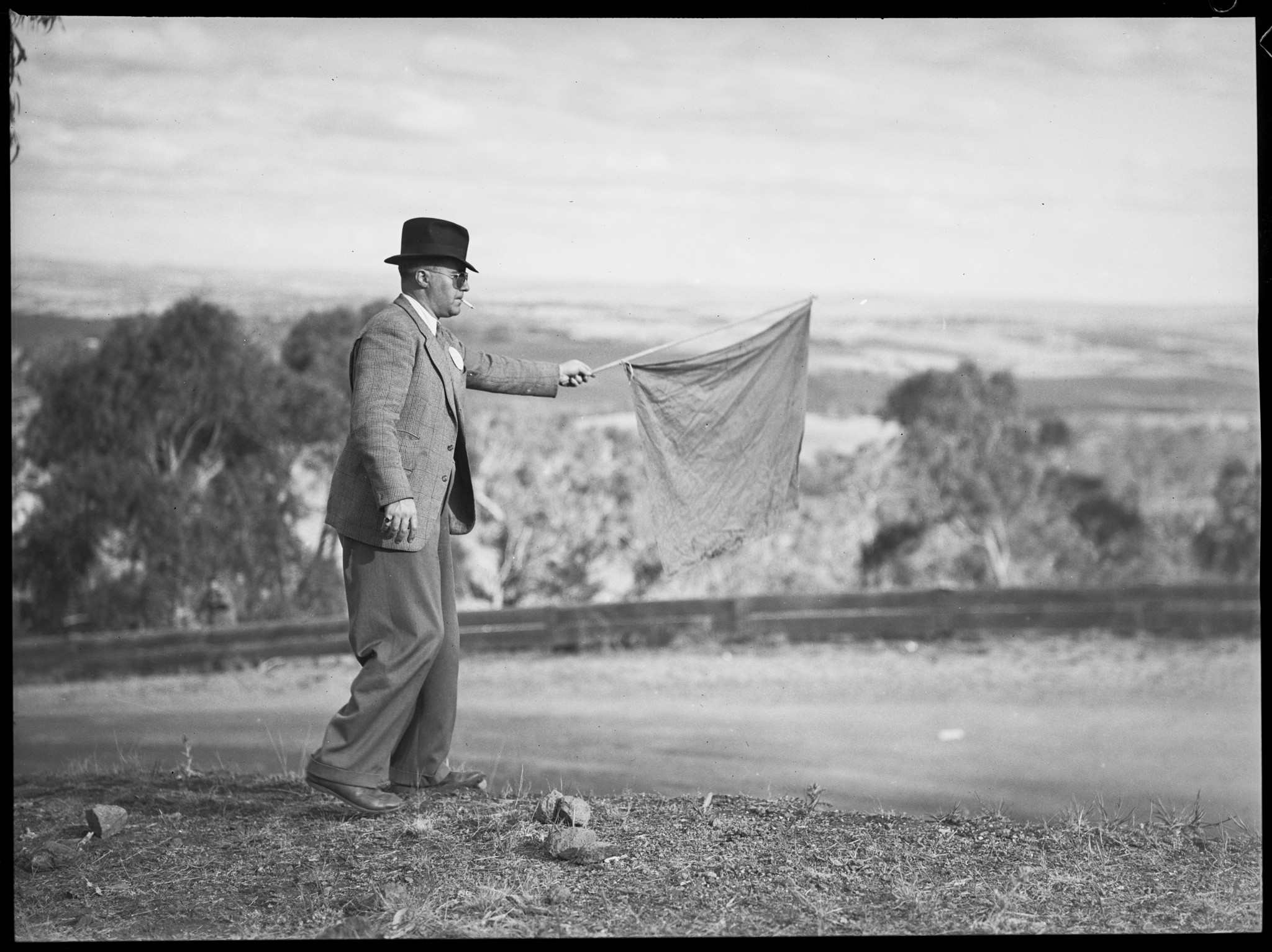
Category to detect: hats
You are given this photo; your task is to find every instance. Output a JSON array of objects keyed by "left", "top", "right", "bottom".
[{"left": 384, "top": 217, "right": 480, "bottom": 274}]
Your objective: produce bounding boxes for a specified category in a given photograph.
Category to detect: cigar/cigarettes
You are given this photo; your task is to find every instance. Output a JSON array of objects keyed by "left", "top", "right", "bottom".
[{"left": 460, "top": 298, "right": 475, "bottom": 309}]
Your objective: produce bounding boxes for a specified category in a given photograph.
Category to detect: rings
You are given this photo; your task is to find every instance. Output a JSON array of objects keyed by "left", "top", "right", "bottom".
[{"left": 384, "top": 517, "right": 392, "bottom": 522}]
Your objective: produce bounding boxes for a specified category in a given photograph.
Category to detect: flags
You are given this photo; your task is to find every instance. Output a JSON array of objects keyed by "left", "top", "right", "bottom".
[{"left": 623, "top": 296, "right": 815, "bottom": 577}]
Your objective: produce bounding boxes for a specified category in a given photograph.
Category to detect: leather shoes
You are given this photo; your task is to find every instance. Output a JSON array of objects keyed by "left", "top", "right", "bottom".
[
  {"left": 304, "top": 772, "right": 405, "bottom": 814},
  {"left": 391, "top": 771, "right": 486, "bottom": 794}
]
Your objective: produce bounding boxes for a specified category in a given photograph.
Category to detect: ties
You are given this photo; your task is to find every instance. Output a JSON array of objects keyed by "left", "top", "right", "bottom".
[{"left": 435, "top": 321, "right": 446, "bottom": 349}]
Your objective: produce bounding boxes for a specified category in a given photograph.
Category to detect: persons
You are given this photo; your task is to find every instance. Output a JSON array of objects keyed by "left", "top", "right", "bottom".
[{"left": 295, "top": 217, "right": 596, "bottom": 816}]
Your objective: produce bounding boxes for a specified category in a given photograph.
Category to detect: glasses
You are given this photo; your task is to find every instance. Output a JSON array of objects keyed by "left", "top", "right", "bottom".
[{"left": 422, "top": 269, "right": 468, "bottom": 288}]
